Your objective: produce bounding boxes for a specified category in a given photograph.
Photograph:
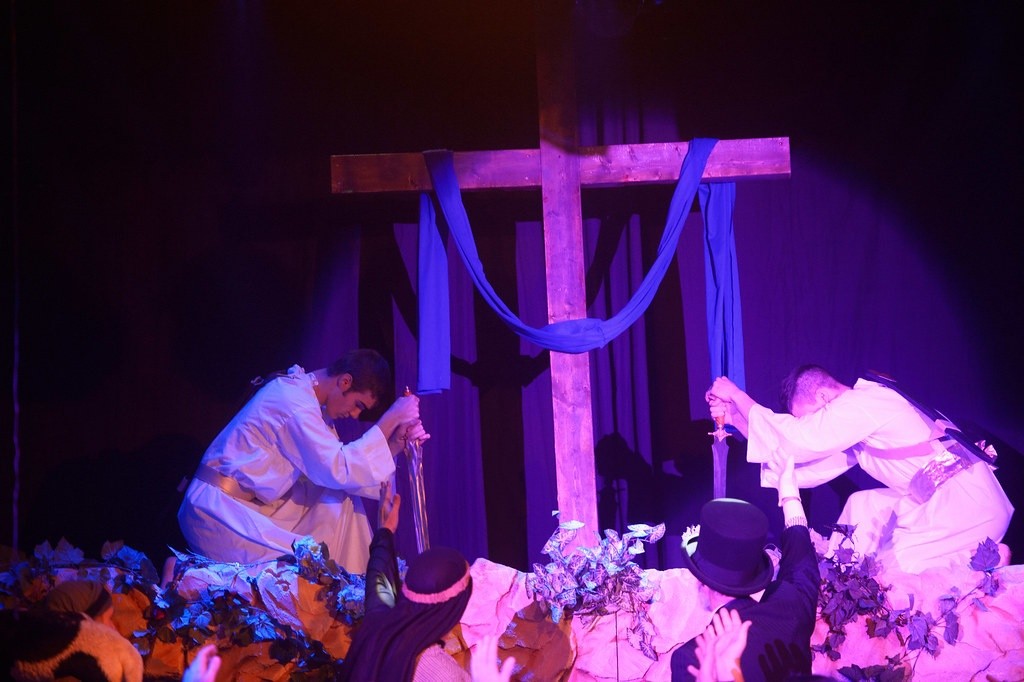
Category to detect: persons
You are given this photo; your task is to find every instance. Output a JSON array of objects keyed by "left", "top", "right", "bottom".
[
  {"left": 161, "top": 347, "right": 432, "bottom": 603},
  {"left": 707, "top": 608, "right": 752, "bottom": 682},
  {"left": 669, "top": 446, "right": 821, "bottom": 682},
  {"left": 38, "top": 580, "right": 222, "bottom": 682},
  {"left": 339, "top": 481, "right": 474, "bottom": 682},
  {"left": 704, "top": 362, "right": 1015, "bottom": 575},
  {"left": 467, "top": 634, "right": 516, "bottom": 682},
  {"left": 688, "top": 628, "right": 716, "bottom": 682}
]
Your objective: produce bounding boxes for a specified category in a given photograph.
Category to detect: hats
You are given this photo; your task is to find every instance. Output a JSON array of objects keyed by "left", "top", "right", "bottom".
[{"left": 680, "top": 497, "right": 774, "bottom": 596}]
